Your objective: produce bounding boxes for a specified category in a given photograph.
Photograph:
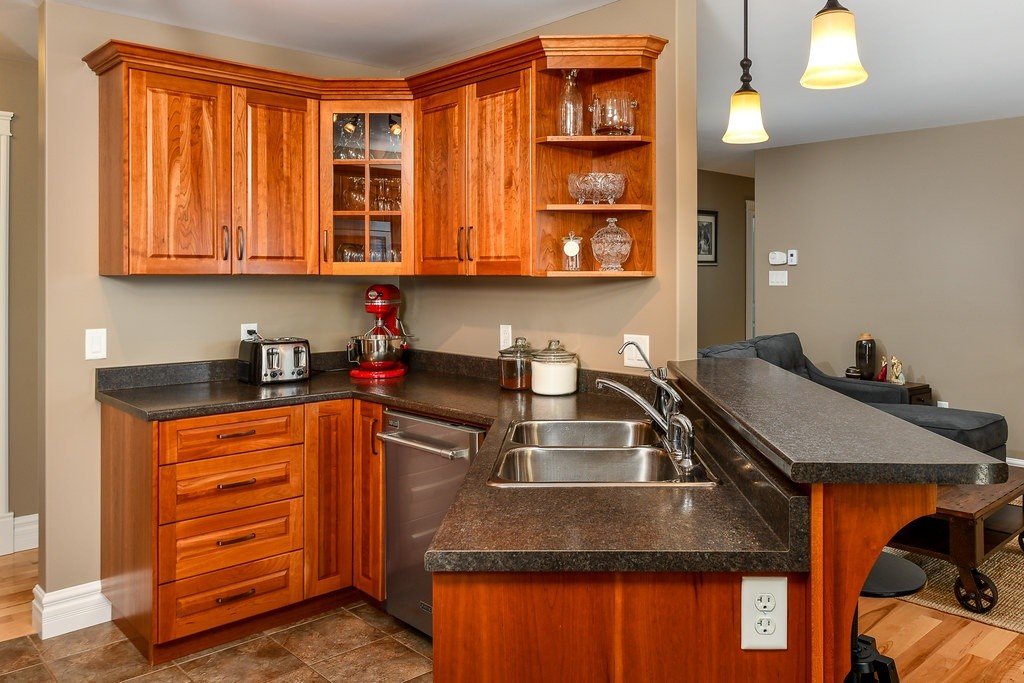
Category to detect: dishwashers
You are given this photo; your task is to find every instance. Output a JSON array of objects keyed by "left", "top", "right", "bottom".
[{"left": 374, "top": 405, "right": 489, "bottom": 638}]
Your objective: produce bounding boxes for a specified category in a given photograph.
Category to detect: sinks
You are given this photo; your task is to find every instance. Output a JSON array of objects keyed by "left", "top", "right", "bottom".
[
  {"left": 485, "top": 448, "right": 721, "bottom": 486},
  {"left": 501, "top": 418, "right": 671, "bottom": 448}
]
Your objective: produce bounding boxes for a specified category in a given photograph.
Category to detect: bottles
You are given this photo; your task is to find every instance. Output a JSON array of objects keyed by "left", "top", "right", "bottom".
[
  {"left": 856, "top": 333, "right": 876, "bottom": 380},
  {"left": 590, "top": 218, "right": 633, "bottom": 271},
  {"left": 530, "top": 340, "right": 579, "bottom": 395},
  {"left": 562, "top": 231, "right": 583, "bottom": 271},
  {"left": 334, "top": 118, "right": 401, "bottom": 159},
  {"left": 558, "top": 69, "right": 583, "bottom": 136},
  {"left": 498, "top": 337, "right": 534, "bottom": 391}
]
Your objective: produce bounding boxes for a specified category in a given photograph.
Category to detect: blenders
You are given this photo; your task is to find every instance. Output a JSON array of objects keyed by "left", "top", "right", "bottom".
[{"left": 347, "top": 285, "right": 413, "bottom": 378}]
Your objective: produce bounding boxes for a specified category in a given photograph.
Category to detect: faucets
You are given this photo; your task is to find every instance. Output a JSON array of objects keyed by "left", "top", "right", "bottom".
[
  {"left": 595, "top": 372, "right": 683, "bottom": 461},
  {"left": 670, "top": 413, "right": 695, "bottom": 468},
  {"left": 618, "top": 340, "right": 668, "bottom": 434}
]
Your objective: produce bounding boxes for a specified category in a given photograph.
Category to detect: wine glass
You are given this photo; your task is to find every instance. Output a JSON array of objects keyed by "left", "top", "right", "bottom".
[{"left": 342, "top": 177, "right": 403, "bottom": 211}]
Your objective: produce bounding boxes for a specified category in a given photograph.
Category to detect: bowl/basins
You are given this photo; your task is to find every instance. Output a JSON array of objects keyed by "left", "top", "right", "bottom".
[
  {"left": 350, "top": 335, "right": 406, "bottom": 370},
  {"left": 568, "top": 173, "right": 625, "bottom": 204}
]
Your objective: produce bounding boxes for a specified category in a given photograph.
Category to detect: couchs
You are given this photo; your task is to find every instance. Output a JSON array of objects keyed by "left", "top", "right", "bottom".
[{"left": 698, "top": 332, "right": 1009, "bottom": 466}]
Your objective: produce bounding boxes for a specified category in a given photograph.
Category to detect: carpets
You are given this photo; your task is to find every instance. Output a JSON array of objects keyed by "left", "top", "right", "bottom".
[{"left": 881, "top": 494, "right": 1024, "bottom": 634}]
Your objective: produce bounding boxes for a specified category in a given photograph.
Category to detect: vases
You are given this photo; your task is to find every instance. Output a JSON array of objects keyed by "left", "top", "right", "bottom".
[{"left": 856, "top": 333, "right": 876, "bottom": 381}]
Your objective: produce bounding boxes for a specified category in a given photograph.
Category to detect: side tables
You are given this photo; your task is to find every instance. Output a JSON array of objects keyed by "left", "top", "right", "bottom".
[{"left": 886, "top": 380, "right": 932, "bottom": 406}]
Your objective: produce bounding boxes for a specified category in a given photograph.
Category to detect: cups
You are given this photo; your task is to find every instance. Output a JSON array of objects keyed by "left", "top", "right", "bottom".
[
  {"left": 590, "top": 91, "right": 637, "bottom": 135},
  {"left": 333, "top": 235, "right": 402, "bottom": 263}
]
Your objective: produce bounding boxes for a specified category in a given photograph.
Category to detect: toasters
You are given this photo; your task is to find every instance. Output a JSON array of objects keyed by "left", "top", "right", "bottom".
[{"left": 232, "top": 336, "right": 311, "bottom": 388}]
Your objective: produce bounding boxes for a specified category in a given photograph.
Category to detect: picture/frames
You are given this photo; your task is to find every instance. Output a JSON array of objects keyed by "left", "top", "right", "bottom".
[{"left": 697, "top": 209, "right": 719, "bottom": 266}]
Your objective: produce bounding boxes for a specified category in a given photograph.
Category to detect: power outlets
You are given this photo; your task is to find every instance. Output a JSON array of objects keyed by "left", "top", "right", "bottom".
[
  {"left": 936, "top": 401, "right": 949, "bottom": 408},
  {"left": 740, "top": 575, "right": 788, "bottom": 651},
  {"left": 240, "top": 323, "right": 258, "bottom": 340},
  {"left": 499, "top": 324, "right": 512, "bottom": 350}
]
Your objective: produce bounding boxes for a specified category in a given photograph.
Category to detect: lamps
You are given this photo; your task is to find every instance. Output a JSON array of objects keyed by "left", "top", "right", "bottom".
[
  {"left": 722, "top": 0, "right": 770, "bottom": 144},
  {"left": 343, "top": 114, "right": 359, "bottom": 134},
  {"left": 388, "top": 114, "right": 401, "bottom": 135},
  {"left": 799, "top": 0, "right": 868, "bottom": 90}
]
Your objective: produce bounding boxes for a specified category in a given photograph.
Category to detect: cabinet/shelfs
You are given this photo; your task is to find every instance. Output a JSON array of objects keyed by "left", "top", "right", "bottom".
[
  {"left": 535, "top": 67, "right": 656, "bottom": 277},
  {"left": 318, "top": 99, "right": 414, "bottom": 276},
  {"left": 159, "top": 404, "right": 304, "bottom": 646},
  {"left": 415, "top": 66, "right": 530, "bottom": 277},
  {"left": 128, "top": 66, "right": 319, "bottom": 277},
  {"left": 354, "top": 395, "right": 385, "bottom": 604},
  {"left": 305, "top": 398, "right": 353, "bottom": 601}
]
